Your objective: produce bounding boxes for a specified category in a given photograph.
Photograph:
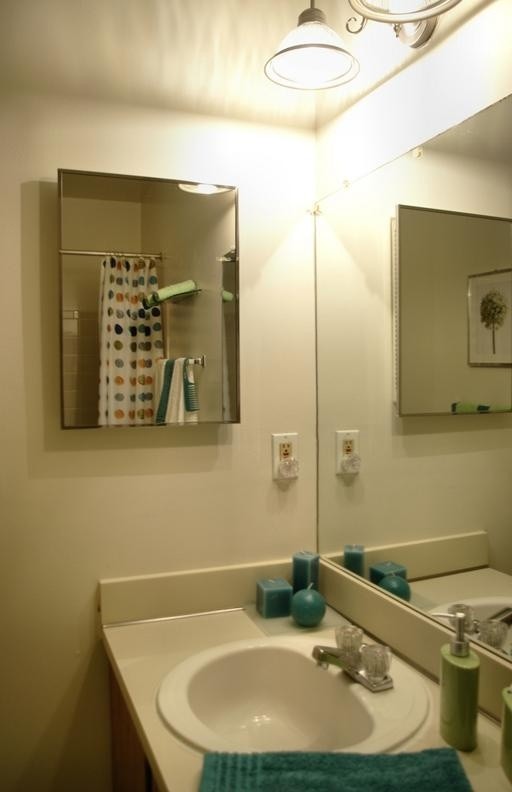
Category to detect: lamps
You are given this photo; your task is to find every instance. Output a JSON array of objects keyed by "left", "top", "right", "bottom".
[{"left": 263, "top": 0, "right": 362, "bottom": 92}]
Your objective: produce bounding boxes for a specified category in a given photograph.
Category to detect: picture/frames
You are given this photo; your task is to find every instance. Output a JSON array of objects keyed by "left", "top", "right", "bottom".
[{"left": 467, "top": 268, "right": 512, "bottom": 367}]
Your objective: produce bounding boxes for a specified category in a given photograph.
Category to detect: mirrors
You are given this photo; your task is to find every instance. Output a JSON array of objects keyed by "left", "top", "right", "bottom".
[
  {"left": 313, "top": 95, "right": 512, "bottom": 735},
  {"left": 394, "top": 202, "right": 512, "bottom": 414},
  {"left": 58, "top": 169, "right": 241, "bottom": 430}
]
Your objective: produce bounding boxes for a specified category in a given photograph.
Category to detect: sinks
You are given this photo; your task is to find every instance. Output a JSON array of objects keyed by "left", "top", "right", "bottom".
[
  {"left": 437, "top": 596, "right": 512, "bottom": 653},
  {"left": 156, "top": 630, "right": 430, "bottom": 755}
]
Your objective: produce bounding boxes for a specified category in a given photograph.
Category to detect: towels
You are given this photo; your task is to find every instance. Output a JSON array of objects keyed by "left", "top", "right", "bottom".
[{"left": 152, "top": 356, "right": 200, "bottom": 425}]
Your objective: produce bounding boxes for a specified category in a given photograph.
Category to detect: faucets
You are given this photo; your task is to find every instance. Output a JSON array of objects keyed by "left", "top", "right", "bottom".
[
  {"left": 313, "top": 642, "right": 351, "bottom": 671},
  {"left": 484, "top": 606, "right": 512, "bottom": 629}
]
